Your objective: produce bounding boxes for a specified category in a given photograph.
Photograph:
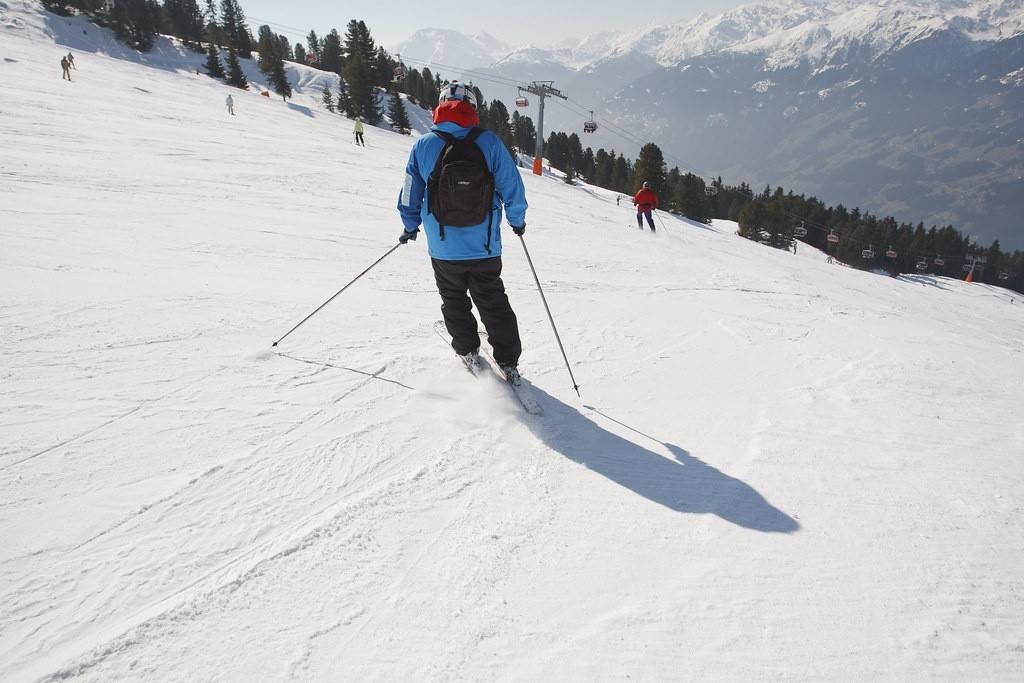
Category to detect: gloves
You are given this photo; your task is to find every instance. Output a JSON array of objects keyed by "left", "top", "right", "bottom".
[
  {"left": 353, "top": 132, "right": 354, "bottom": 133},
  {"left": 399, "top": 227, "right": 421, "bottom": 243},
  {"left": 509, "top": 221, "right": 527, "bottom": 236}
]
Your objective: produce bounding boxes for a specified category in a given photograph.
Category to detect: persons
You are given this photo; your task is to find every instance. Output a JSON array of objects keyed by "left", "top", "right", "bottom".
[
  {"left": 634, "top": 181, "right": 658, "bottom": 233},
  {"left": 397, "top": 80, "right": 529, "bottom": 386},
  {"left": 353, "top": 117, "right": 364, "bottom": 147},
  {"left": 226, "top": 94, "right": 234, "bottom": 115},
  {"left": 617, "top": 195, "right": 621, "bottom": 205},
  {"left": 61, "top": 56, "right": 71, "bottom": 81},
  {"left": 794, "top": 242, "right": 797, "bottom": 254},
  {"left": 68, "top": 52, "right": 75, "bottom": 69}
]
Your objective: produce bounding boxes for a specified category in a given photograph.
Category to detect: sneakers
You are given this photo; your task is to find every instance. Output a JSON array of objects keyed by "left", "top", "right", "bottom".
[
  {"left": 500, "top": 366, "right": 521, "bottom": 386},
  {"left": 455, "top": 347, "right": 480, "bottom": 364}
]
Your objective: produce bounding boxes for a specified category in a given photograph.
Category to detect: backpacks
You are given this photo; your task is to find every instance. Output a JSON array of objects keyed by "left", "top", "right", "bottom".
[{"left": 428, "top": 126, "right": 492, "bottom": 255}]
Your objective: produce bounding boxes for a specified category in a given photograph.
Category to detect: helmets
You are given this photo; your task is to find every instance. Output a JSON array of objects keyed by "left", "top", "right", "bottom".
[
  {"left": 438, "top": 81, "right": 477, "bottom": 110},
  {"left": 355, "top": 117, "right": 360, "bottom": 122},
  {"left": 643, "top": 181, "right": 650, "bottom": 187}
]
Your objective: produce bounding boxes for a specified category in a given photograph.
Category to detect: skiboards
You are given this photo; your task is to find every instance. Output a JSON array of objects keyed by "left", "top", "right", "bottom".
[{"left": 430, "top": 320, "right": 546, "bottom": 415}]
